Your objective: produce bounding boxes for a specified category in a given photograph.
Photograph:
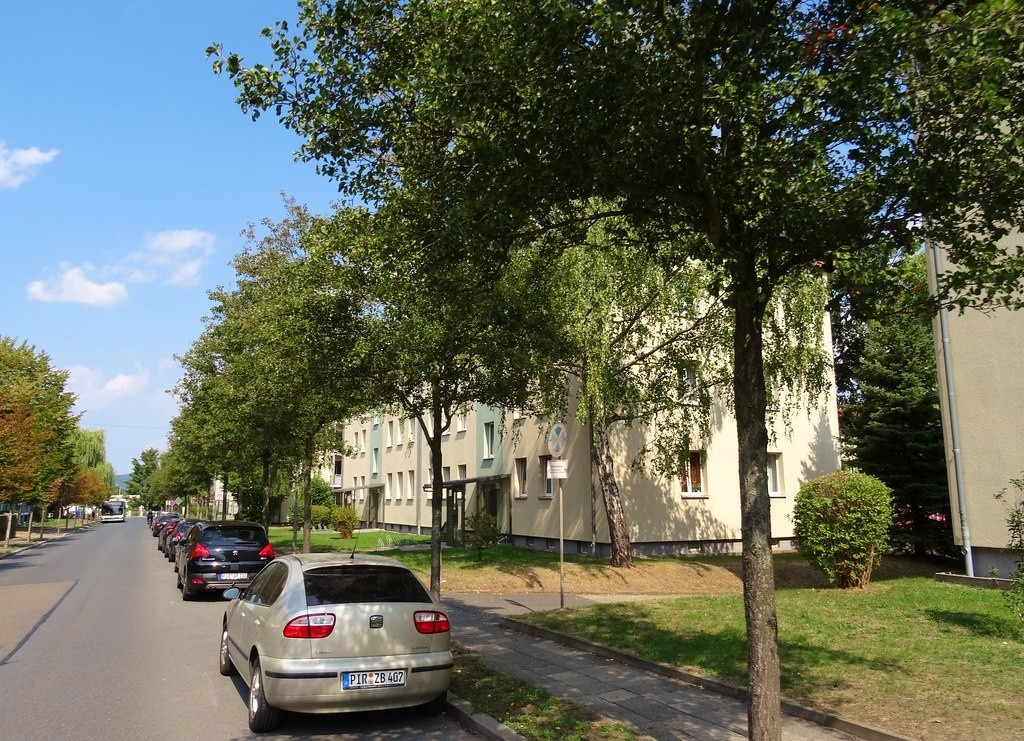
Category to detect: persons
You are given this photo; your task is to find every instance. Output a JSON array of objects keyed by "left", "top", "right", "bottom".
[
  {"left": 92, "top": 510, "right": 95, "bottom": 520},
  {"left": 146, "top": 509, "right": 155, "bottom": 526}
]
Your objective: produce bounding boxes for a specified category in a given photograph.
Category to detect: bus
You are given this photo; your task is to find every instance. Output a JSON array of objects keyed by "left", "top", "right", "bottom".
[{"left": 99, "top": 500, "right": 127, "bottom": 522}]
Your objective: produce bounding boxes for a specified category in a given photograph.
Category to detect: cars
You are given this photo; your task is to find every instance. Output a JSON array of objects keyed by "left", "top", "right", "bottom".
[
  {"left": 171, "top": 522, "right": 276, "bottom": 602},
  {"left": 152, "top": 513, "right": 208, "bottom": 573},
  {"left": 219, "top": 551, "right": 455, "bottom": 734}
]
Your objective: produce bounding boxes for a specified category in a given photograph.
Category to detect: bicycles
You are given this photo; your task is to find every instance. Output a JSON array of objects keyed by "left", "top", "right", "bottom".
[{"left": 147, "top": 515, "right": 154, "bottom": 529}]
